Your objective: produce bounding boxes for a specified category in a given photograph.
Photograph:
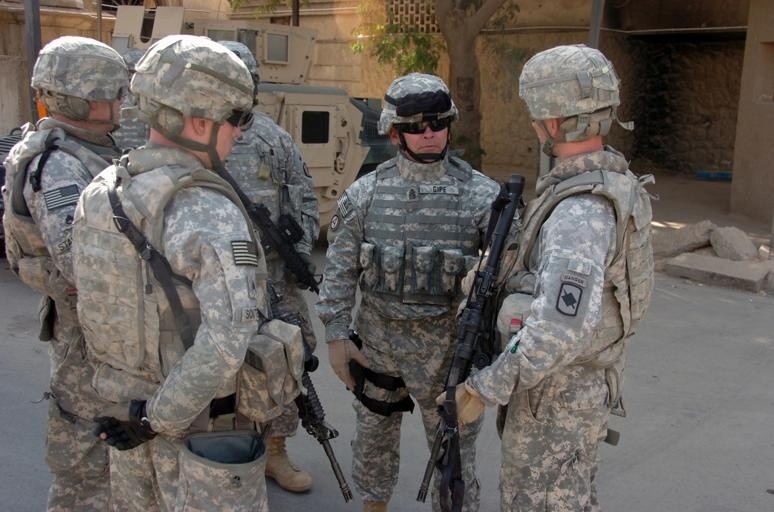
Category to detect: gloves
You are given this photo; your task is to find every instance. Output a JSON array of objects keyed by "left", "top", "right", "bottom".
[
  {"left": 328, "top": 338, "right": 370, "bottom": 392},
  {"left": 435, "top": 377, "right": 487, "bottom": 423},
  {"left": 91, "top": 400, "right": 161, "bottom": 451}
]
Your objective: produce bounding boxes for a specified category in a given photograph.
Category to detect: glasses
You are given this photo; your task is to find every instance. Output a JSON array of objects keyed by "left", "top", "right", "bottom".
[
  {"left": 396, "top": 119, "right": 449, "bottom": 133},
  {"left": 225, "top": 108, "right": 254, "bottom": 124}
]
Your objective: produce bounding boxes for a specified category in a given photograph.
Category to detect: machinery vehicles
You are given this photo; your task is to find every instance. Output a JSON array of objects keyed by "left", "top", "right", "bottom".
[{"left": 1, "top": 7, "right": 468, "bottom": 247}]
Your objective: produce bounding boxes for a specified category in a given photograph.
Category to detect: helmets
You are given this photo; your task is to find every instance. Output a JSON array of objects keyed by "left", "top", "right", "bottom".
[
  {"left": 518, "top": 44, "right": 621, "bottom": 119},
  {"left": 376, "top": 73, "right": 459, "bottom": 134},
  {"left": 130, "top": 33, "right": 255, "bottom": 123},
  {"left": 219, "top": 40, "right": 260, "bottom": 79},
  {"left": 31, "top": 35, "right": 126, "bottom": 101}
]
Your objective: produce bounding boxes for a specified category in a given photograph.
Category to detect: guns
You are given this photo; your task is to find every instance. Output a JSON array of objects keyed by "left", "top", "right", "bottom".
[
  {"left": 216, "top": 167, "right": 319, "bottom": 295},
  {"left": 418, "top": 174, "right": 527, "bottom": 503},
  {"left": 265, "top": 281, "right": 355, "bottom": 503}
]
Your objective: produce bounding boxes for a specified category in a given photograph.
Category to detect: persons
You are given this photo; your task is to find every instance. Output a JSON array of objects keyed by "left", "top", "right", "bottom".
[
  {"left": 2, "top": 35, "right": 131, "bottom": 510},
  {"left": 113, "top": 47, "right": 149, "bottom": 154},
  {"left": 216, "top": 40, "right": 320, "bottom": 491},
  {"left": 72, "top": 36, "right": 308, "bottom": 511},
  {"left": 437, "top": 45, "right": 658, "bottom": 512},
  {"left": 314, "top": 68, "right": 522, "bottom": 511}
]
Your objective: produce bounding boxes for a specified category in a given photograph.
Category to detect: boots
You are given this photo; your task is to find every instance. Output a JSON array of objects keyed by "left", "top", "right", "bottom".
[{"left": 263, "top": 436, "right": 315, "bottom": 491}]
